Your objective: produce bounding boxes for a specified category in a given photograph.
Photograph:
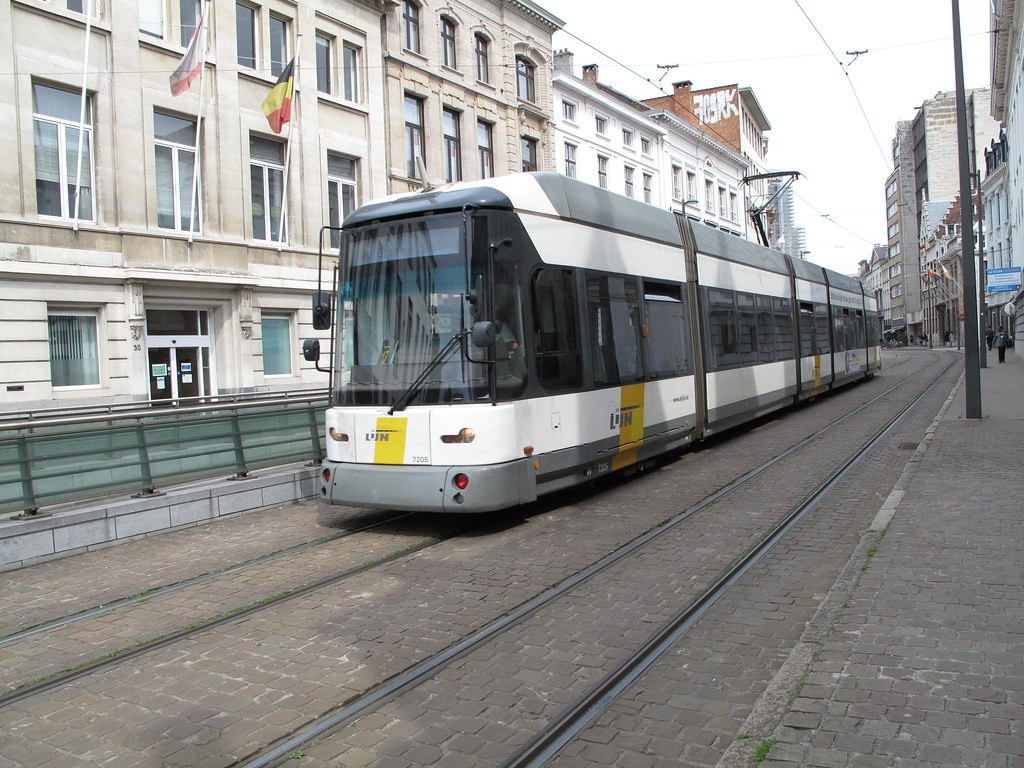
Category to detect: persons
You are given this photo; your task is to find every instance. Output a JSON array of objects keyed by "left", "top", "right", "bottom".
[
  {"left": 985, "top": 326, "right": 994, "bottom": 351},
  {"left": 993, "top": 326, "right": 1008, "bottom": 363},
  {"left": 918, "top": 333, "right": 928, "bottom": 347},
  {"left": 493, "top": 304, "right": 518, "bottom": 350},
  {"left": 944, "top": 331, "right": 954, "bottom": 348}
]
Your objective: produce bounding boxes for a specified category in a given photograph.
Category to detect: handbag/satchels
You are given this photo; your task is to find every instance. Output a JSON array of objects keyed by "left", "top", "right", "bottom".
[{"left": 1006, "top": 338, "right": 1014, "bottom": 348}]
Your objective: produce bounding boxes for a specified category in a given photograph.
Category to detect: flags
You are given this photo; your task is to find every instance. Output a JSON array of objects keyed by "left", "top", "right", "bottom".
[
  {"left": 928, "top": 269, "right": 941, "bottom": 281},
  {"left": 169, "top": 14, "right": 205, "bottom": 97},
  {"left": 261, "top": 57, "right": 296, "bottom": 135},
  {"left": 941, "top": 265, "right": 951, "bottom": 280}
]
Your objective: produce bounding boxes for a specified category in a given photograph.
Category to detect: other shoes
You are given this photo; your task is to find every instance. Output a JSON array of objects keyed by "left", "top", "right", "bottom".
[
  {"left": 988, "top": 347, "right": 992, "bottom": 351},
  {"left": 998, "top": 360, "right": 1005, "bottom": 364}
]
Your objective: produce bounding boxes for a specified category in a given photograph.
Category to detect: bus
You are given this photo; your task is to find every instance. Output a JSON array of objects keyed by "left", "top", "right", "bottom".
[{"left": 301, "top": 169, "right": 881, "bottom": 514}]
